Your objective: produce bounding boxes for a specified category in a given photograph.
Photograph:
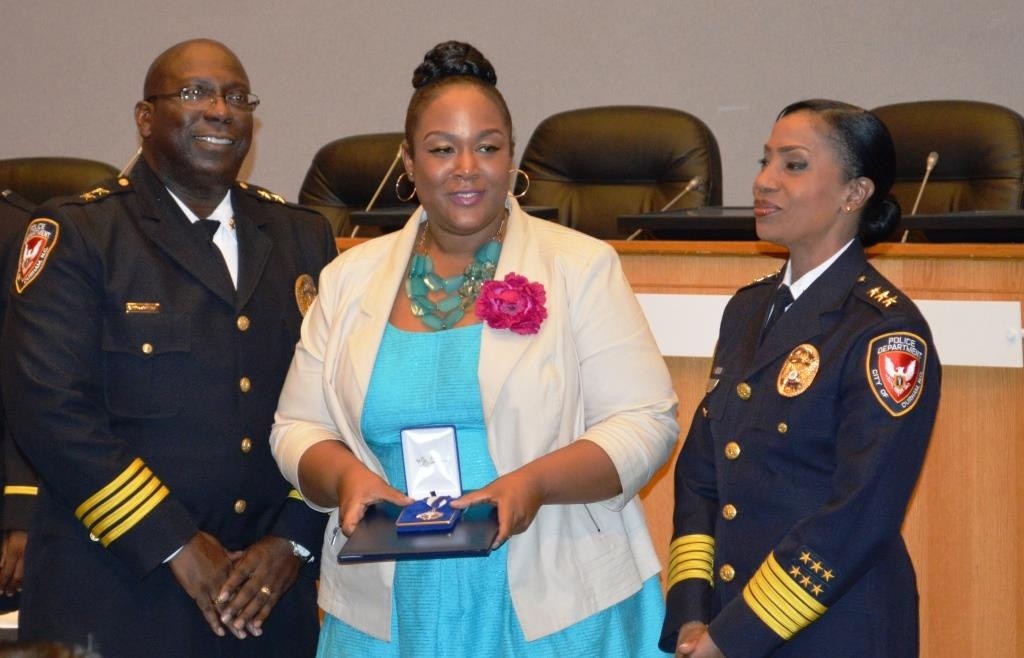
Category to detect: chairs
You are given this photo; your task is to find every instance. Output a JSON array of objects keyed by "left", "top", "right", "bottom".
[{"left": 0, "top": 97, "right": 1024, "bottom": 253}]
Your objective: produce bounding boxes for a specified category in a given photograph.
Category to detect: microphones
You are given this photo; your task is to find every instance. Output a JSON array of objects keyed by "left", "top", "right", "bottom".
[
  {"left": 350, "top": 139, "right": 407, "bottom": 237},
  {"left": 628, "top": 177, "right": 702, "bottom": 240},
  {"left": 900, "top": 151, "right": 938, "bottom": 242}
]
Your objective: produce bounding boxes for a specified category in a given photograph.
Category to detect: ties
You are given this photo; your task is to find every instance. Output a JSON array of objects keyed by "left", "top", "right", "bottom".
[
  {"left": 194, "top": 217, "right": 234, "bottom": 290},
  {"left": 760, "top": 283, "right": 794, "bottom": 346}
]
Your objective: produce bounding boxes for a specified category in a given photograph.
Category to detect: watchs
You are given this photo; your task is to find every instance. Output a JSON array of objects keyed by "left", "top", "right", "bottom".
[{"left": 290, "top": 541, "right": 312, "bottom": 560}]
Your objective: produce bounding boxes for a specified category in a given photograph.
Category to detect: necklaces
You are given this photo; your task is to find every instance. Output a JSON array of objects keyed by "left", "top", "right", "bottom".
[{"left": 404, "top": 222, "right": 504, "bottom": 329}]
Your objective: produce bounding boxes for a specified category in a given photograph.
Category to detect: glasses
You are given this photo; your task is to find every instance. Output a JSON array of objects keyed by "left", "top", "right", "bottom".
[{"left": 144, "top": 85, "right": 262, "bottom": 114}]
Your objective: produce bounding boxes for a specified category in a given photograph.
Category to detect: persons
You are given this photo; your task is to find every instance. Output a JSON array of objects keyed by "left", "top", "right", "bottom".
[
  {"left": 0, "top": 38, "right": 337, "bottom": 658},
  {"left": 657, "top": 96, "right": 943, "bottom": 658},
  {"left": 267, "top": 39, "right": 678, "bottom": 658}
]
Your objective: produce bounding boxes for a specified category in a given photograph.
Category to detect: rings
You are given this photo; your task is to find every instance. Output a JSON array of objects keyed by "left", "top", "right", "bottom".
[{"left": 261, "top": 585, "right": 272, "bottom": 595}]
